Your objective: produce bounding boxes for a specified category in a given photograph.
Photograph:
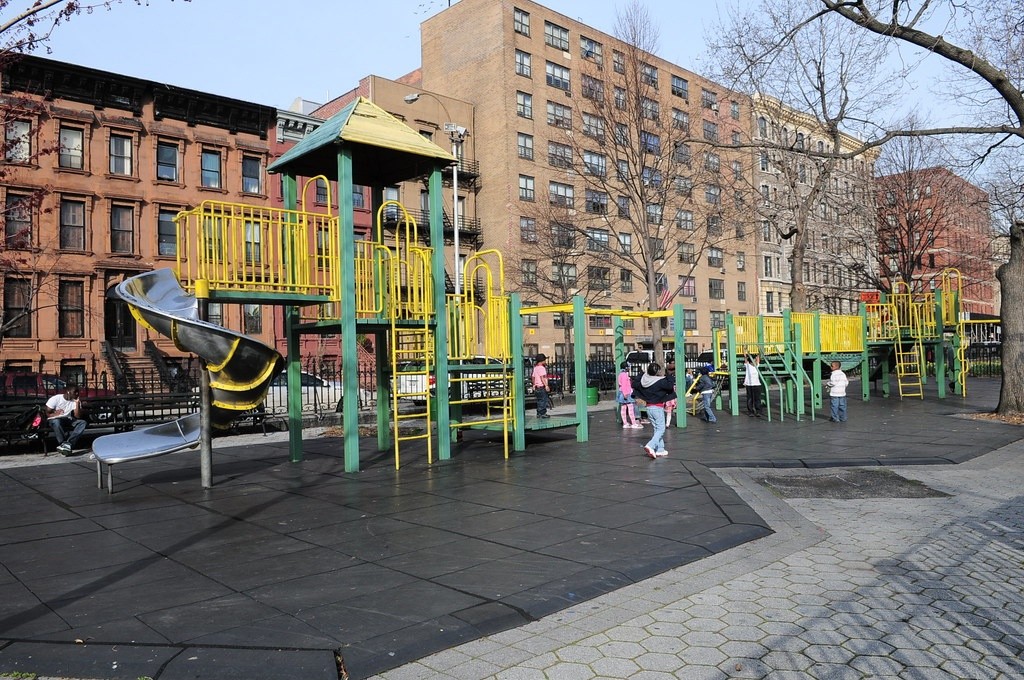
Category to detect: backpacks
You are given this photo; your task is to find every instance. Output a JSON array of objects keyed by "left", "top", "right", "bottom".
[{"left": 21, "top": 410, "right": 47, "bottom": 439}]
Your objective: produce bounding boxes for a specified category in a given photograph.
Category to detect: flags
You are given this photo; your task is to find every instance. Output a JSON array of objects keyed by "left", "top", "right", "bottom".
[{"left": 659, "top": 285, "right": 674, "bottom": 309}]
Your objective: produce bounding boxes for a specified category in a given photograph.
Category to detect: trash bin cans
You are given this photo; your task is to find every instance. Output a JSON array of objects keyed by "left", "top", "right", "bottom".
[
  {"left": 425, "top": 386, "right": 453, "bottom": 421},
  {"left": 587, "top": 386, "right": 599, "bottom": 406}
]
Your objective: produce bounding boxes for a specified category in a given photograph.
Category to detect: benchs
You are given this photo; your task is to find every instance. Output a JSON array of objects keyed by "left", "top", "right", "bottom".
[{"left": 0, "top": 392, "right": 282, "bottom": 458}]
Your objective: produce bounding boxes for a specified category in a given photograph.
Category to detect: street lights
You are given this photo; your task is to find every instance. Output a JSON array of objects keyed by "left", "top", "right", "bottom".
[{"left": 403, "top": 92, "right": 467, "bottom": 302}]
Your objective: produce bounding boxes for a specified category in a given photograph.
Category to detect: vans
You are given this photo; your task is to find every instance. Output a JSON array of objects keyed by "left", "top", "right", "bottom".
[
  {"left": 693, "top": 348, "right": 728, "bottom": 374},
  {"left": 626, "top": 349, "right": 689, "bottom": 380}
]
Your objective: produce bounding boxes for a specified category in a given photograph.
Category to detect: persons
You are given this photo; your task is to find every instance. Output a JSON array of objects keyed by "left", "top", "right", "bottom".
[
  {"left": 823, "top": 361, "right": 848, "bottom": 422},
  {"left": 631, "top": 362, "right": 677, "bottom": 459},
  {"left": 641, "top": 358, "right": 717, "bottom": 428},
  {"left": 616, "top": 361, "right": 644, "bottom": 429},
  {"left": 532, "top": 354, "right": 550, "bottom": 418},
  {"left": 744, "top": 348, "right": 761, "bottom": 417},
  {"left": 46, "top": 383, "right": 87, "bottom": 457}
]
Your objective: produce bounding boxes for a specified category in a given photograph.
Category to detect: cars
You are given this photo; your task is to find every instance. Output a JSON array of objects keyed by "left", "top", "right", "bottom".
[
  {"left": 400, "top": 356, "right": 508, "bottom": 407},
  {"left": 523, "top": 359, "right": 615, "bottom": 394},
  {"left": 188, "top": 369, "right": 343, "bottom": 417},
  {"left": 0, "top": 370, "right": 123, "bottom": 422}
]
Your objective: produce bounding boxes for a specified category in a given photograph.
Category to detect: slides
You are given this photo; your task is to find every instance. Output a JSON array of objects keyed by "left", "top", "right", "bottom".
[{"left": 91, "top": 265, "right": 289, "bottom": 468}]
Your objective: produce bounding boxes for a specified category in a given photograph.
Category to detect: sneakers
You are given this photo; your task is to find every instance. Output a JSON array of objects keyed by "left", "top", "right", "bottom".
[
  {"left": 57, "top": 443, "right": 72, "bottom": 456},
  {"left": 655, "top": 450, "right": 668, "bottom": 456},
  {"left": 644, "top": 446, "right": 656, "bottom": 459}
]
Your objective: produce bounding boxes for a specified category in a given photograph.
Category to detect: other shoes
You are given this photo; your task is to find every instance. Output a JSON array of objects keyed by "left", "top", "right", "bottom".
[
  {"left": 537, "top": 414, "right": 550, "bottom": 418},
  {"left": 749, "top": 412, "right": 754, "bottom": 417},
  {"left": 623, "top": 422, "right": 631, "bottom": 428},
  {"left": 705, "top": 419, "right": 717, "bottom": 424},
  {"left": 756, "top": 412, "right": 762, "bottom": 417},
  {"left": 829, "top": 418, "right": 834, "bottom": 422},
  {"left": 631, "top": 423, "right": 644, "bottom": 428},
  {"left": 665, "top": 423, "right": 670, "bottom": 429},
  {"left": 641, "top": 418, "right": 650, "bottom": 424}
]
